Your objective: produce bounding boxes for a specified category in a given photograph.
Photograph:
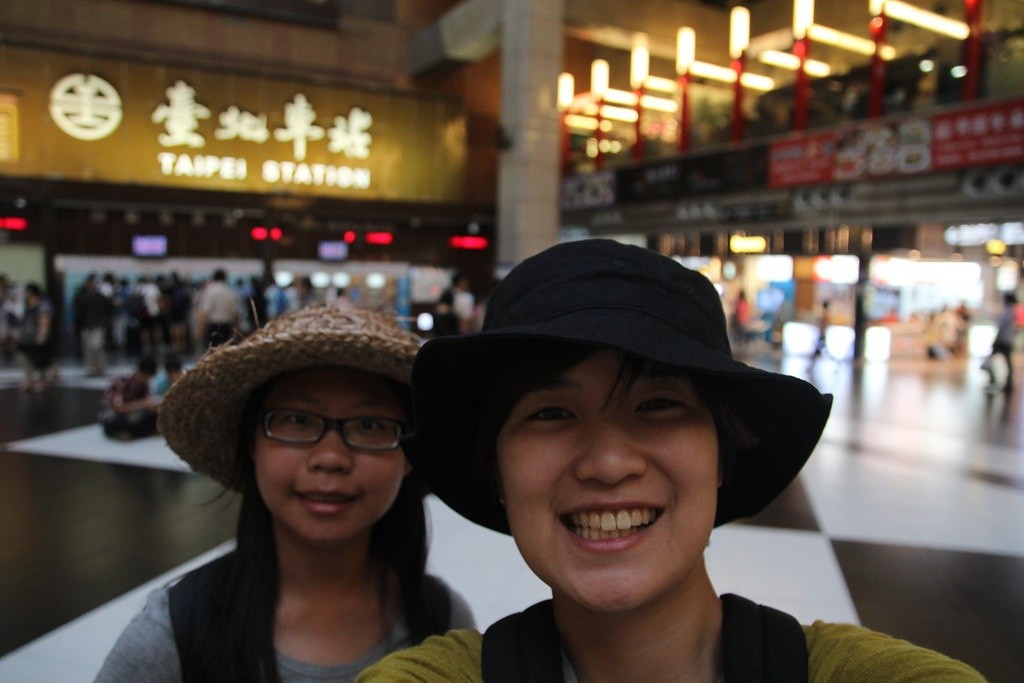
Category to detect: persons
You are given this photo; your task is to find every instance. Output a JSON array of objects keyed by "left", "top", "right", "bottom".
[
  {"left": 93, "top": 305, "right": 480, "bottom": 682},
  {"left": 353, "top": 237, "right": 989, "bottom": 683},
  {"left": 810, "top": 300, "right": 830, "bottom": 358},
  {"left": 1, "top": 266, "right": 492, "bottom": 442},
  {"left": 734, "top": 290, "right": 751, "bottom": 343},
  {"left": 987, "top": 293, "right": 1019, "bottom": 393}
]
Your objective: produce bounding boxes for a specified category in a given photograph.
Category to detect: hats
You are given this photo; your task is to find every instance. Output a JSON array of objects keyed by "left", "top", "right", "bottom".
[
  {"left": 397, "top": 237, "right": 833, "bottom": 537},
  {"left": 158, "top": 302, "right": 428, "bottom": 497}
]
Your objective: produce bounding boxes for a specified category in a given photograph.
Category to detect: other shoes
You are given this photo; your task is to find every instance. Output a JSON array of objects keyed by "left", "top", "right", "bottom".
[
  {"left": 983, "top": 384, "right": 995, "bottom": 391},
  {"left": 1002, "top": 385, "right": 1012, "bottom": 392}
]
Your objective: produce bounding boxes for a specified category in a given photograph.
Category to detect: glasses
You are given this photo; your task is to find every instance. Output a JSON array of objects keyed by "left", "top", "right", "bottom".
[{"left": 253, "top": 404, "right": 416, "bottom": 454}]
